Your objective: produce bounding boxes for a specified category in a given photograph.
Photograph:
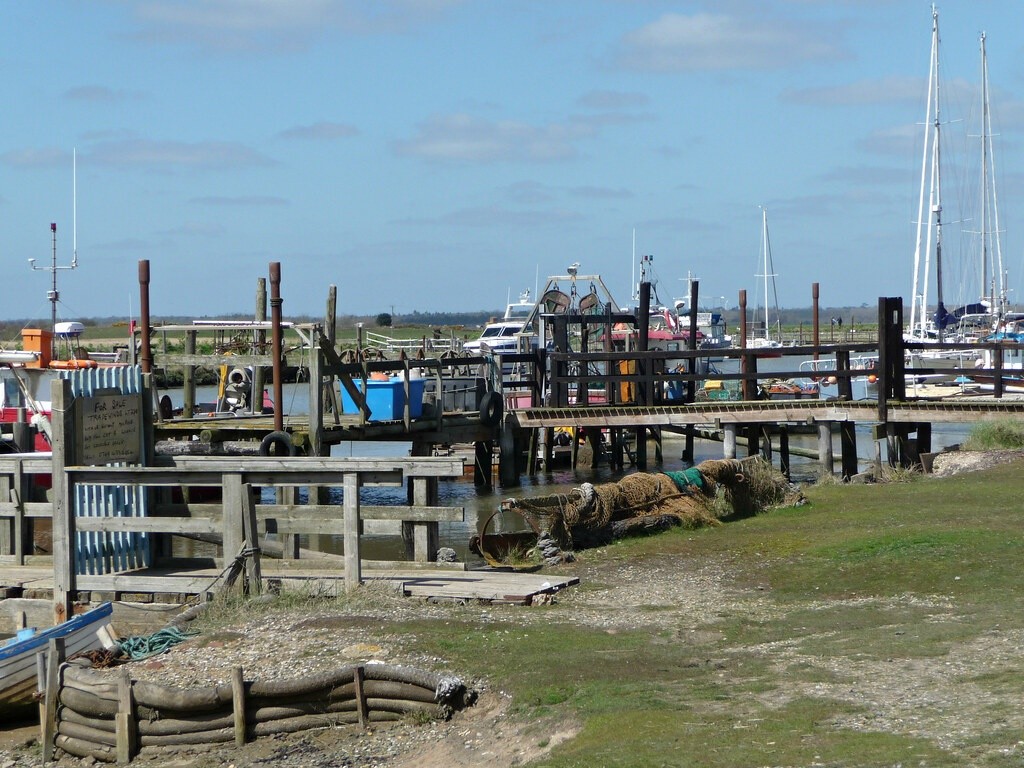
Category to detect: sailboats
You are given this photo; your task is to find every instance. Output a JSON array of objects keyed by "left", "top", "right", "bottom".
[{"left": 903, "top": 2, "right": 1024, "bottom": 387}]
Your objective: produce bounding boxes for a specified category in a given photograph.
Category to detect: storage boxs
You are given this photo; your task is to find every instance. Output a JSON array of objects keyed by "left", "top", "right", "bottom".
[
  {"left": 768, "top": 391, "right": 801, "bottom": 400},
  {"left": 338, "top": 376, "right": 399, "bottom": 415},
  {"left": 801, "top": 393, "right": 819, "bottom": 399},
  {"left": 359, "top": 378, "right": 427, "bottom": 421}
]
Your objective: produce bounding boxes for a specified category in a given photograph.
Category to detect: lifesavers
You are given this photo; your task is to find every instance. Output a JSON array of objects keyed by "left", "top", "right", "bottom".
[
  {"left": 228, "top": 368, "right": 248, "bottom": 388},
  {"left": 48, "top": 358, "right": 98, "bottom": 369},
  {"left": 259, "top": 430, "right": 300, "bottom": 457},
  {"left": 479, "top": 390, "right": 504, "bottom": 427}
]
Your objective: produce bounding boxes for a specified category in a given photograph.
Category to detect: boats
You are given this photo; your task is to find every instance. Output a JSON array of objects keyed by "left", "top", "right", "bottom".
[
  {"left": 367, "top": 204, "right": 819, "bottom": 465},
  {"left": 0, "top": 602, "right": 120, "bottom": 706},
  {"left": 0, "top": 146, "right": 272, "bottom": 502}
]
